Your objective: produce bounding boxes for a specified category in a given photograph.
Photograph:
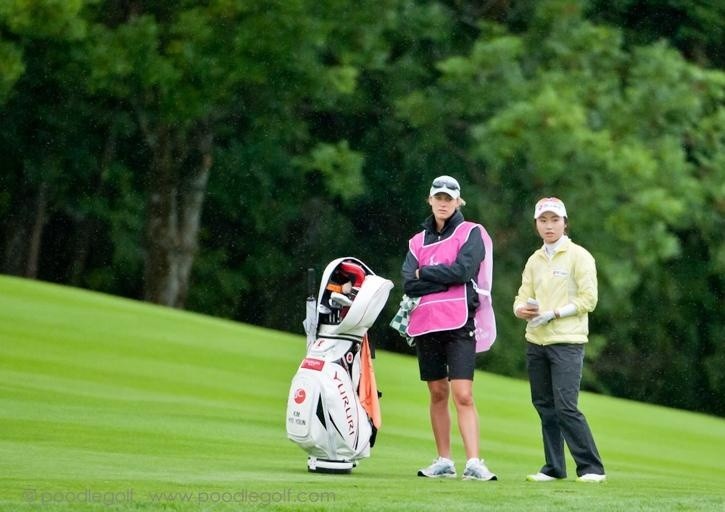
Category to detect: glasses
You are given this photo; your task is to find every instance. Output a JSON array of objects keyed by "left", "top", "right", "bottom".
[{"left": 432, "top": 181, "right": 459, "bottom": 191}]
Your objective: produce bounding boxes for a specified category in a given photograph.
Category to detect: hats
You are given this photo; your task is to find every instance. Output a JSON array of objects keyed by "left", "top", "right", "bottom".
[
  {"left": 430, "top": 175, "right": 460, "bottom": 199},
  {"left": 533, "top": 197, "right": 569, "bottom": 220}
]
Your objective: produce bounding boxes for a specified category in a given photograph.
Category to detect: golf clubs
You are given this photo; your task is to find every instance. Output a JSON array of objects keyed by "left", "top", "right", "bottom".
[{"left": 318, "top": 262, "right": 365, "bottom": 325}]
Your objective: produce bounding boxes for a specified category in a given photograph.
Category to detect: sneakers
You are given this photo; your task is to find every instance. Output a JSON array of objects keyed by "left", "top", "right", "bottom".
[
  {"left": 462, "top": 459, "right": 497, "bottom": 480},
  {"left": 575, "top": 473, "right": 604, "bottom": 483},
  {"left": 417, "top": 459, "right": 456, "bottom": 477},
  {"left": 527, "top": 471, "right": 554, "bottom": 482}
]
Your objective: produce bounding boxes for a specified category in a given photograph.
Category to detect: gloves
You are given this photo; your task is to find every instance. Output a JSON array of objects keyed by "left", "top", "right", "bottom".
[{"left": 530, "top": 309, "right": 556, "bottom": 328}]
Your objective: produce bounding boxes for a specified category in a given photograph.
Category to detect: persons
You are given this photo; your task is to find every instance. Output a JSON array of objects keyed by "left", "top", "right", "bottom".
[
  {"left": 400, "top": 174, "right": 500, "bottom": 482},
  {"left": 512, "top": 195, "right": 607, "bottom": 484}
]
